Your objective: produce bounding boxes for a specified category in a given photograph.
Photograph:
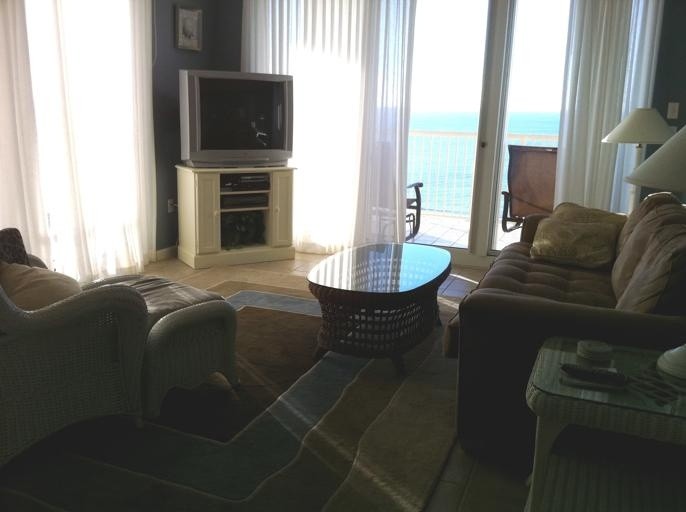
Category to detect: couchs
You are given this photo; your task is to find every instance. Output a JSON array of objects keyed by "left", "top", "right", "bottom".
[
  {"left": 0, "top": 226, "right": 152, "bottom": 471},
  {"left": 78, "top": 268, "right": 240, "bottom": 428},
  {"left": 439, "top": 188, "right": 686, "bottom": 479}
]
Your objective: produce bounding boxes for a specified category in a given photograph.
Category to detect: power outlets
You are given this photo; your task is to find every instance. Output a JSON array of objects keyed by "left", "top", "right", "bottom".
[{"left": 166, "top": 198, "right": 175, "bottom": 215}]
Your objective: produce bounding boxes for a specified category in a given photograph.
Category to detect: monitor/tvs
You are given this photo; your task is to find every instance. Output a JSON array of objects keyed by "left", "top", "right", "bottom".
[{"left": 179, "top": 70, "right": 293, "bottom": 168}]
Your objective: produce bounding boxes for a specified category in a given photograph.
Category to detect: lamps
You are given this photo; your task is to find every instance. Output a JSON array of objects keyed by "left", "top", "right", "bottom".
[
  {"left": 598, "top": 106, "right": 679, "bottom": 217},
  {"left": 620, "top": 122, "right": 686, "bottom": 382}
]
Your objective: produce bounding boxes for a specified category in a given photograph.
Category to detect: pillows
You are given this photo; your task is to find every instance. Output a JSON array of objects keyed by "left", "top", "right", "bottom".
[
  {"left": 546, "top": 201, "right": 629, "bottom": 230},
  {"left": 530, "top": 217, "right": 620, "bottom": 272},
  {"left": 0, "top": 260, "right": 83, "bottom": 312}
]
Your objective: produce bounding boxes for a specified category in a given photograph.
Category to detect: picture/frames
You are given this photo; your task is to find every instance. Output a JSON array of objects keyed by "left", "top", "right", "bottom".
[{"left": 172, "top": 2, "right": 204, "bottom": 54}]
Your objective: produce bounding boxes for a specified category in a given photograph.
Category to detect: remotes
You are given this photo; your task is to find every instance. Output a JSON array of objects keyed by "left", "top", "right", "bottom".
[{"left": 562, "top": 364, "right": 634, "bottom": 386}]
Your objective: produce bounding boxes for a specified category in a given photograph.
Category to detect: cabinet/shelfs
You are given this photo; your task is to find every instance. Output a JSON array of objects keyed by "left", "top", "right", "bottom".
[{"left": 173, "top": 162, "right": 297, "bottom": 271}]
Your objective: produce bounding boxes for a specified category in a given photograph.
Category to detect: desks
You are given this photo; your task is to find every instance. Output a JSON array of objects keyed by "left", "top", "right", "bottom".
[{"left": 521, "top": 334, "right": 686, "bottom": 512}]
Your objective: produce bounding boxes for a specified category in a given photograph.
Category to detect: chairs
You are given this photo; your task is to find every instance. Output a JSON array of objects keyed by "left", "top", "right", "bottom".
[
  {"left": 403, "top": 181, "right": 425, "bottom": 243},
  {"left": 498, "top": 143, "right": 559, "bottom": 244}
]
